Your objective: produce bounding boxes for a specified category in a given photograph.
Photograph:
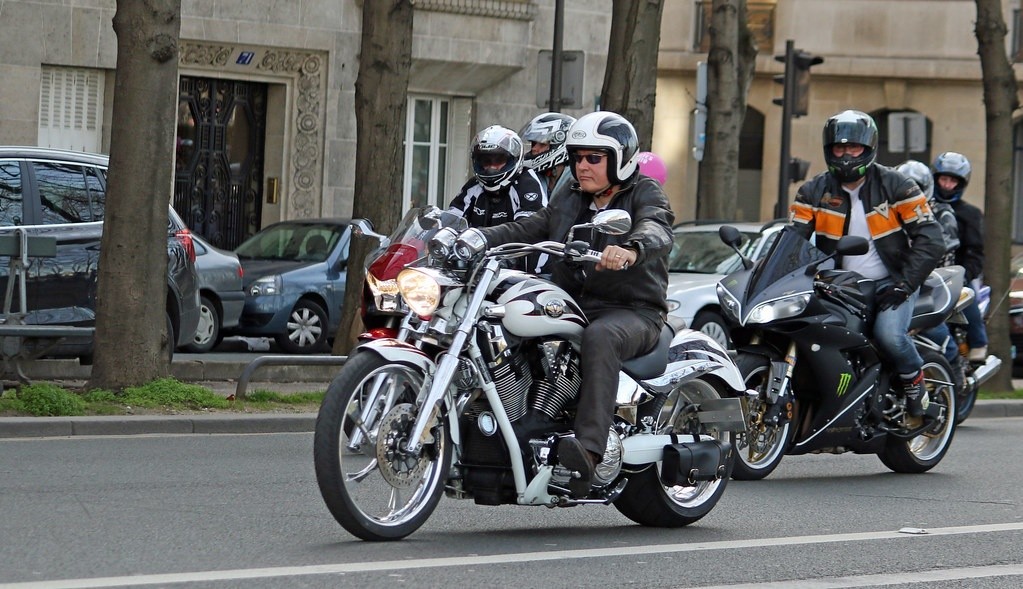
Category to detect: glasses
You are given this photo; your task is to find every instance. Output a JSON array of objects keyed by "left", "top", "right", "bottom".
[
  {"left": 833, "top": 144, "right": 862, "bottom": 152},
  {"left": 571, "top": 152, "right": 606, "bottom": 164}
]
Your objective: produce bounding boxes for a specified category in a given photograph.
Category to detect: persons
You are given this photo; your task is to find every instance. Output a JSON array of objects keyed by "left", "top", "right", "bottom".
[
  {"left": 787, "top": 109, "right": 946, "bottom": 417},
  {"left": 477, "top": 111, "right": 675, "bottom": 482},
  {"left": 894, "top": 159, "right": 967, "bottom": 394},
  {"left": 415, "top": 125, "right": 550, "bottom": 274},
  {"left": 930, "top": 151, "right": 990, "bottom": 360},
  {"left": 518, "top": 112, "right": 574, "bottom": 203}
]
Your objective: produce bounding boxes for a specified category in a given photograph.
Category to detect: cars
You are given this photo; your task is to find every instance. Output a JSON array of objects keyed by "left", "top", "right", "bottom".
[
  {"left": 169, "top": 201, "right": 246, "bottom": 354},
  {"left": 235, "top": 218, "right": 379, "bottom": 353}
]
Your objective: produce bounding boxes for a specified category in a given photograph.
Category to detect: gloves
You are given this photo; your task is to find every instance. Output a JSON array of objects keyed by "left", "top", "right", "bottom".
[{"left": 874, "top": 285, "right": 907, "bottom": 311}]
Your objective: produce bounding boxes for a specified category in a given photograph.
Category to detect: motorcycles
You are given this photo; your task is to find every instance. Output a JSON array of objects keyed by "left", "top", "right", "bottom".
[{"left": 314, "top": 195, "right": 1002, "bottom": 543}]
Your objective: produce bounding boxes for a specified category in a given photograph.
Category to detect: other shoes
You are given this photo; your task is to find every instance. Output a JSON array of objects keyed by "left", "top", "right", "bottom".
[
  {"left": 948, "top": 352, "right": 965, "bottom": 394},
  {"left": 554, "top": 438, "right": 596, "bottom": 498},
  {"left": 968, "top": 345, "right": 987, "bottom": 359},
  {"left": 901, "top": 368, "right": 929, "bottom": 417}
]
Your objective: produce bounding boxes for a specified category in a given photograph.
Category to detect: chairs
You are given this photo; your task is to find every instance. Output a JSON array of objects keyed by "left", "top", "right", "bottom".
[{"left": 306, "top": 236, "right": 327, "bottom": 254}]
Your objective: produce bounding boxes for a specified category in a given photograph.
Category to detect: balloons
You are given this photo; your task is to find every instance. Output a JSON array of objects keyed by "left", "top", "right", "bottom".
[{"left": 638, "top": 152, "right": 667, "bottom": 185}]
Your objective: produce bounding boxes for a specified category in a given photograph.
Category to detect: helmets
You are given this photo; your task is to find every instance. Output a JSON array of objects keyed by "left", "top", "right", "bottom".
[
  {"left": 471, "top": 125, "right": 525, "bottom": 192},
  {"left": 522, "top": 113, "right": 577, "bottom": 173},
  {"left": 566, "top": 111, "right": 640, "bottom": 183},
  {"left": 638, "top": 151, "right": 667, "bottom": 186},
  {"left": 892, "top": 160, "right": 934, "bottom": 203},
  {"left": 930, "top": 152, "right": 971, "bottom": 202},
  {"left": 822, "top": 110, "right": 879, "bottom": 182}
]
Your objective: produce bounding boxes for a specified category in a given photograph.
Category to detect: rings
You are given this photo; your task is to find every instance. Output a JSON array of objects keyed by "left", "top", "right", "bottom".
[{"left": 616, "top": 255, "right": 620, "bottom": 258}]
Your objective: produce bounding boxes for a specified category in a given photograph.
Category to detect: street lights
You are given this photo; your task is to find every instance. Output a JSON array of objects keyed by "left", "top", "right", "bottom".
[
  {"left": 792, "top": 49, "right": 824, "bottom": 119},
  {"left": 772, "top": 53, "right": 788, "bottom": 108}
]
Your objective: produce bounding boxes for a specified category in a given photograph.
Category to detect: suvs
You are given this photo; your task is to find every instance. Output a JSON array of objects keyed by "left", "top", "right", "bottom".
[
  {"left": 1, "top": 146, "right": 201, "bottom": 364},
  {"left": 662, "top": 217, "right": 788, "bottom": 352}
]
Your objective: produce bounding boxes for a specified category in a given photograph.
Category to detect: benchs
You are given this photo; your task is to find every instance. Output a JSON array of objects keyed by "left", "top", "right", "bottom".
[{"left": 0, "top": 228, "right": 94, "bottom": 389}]
